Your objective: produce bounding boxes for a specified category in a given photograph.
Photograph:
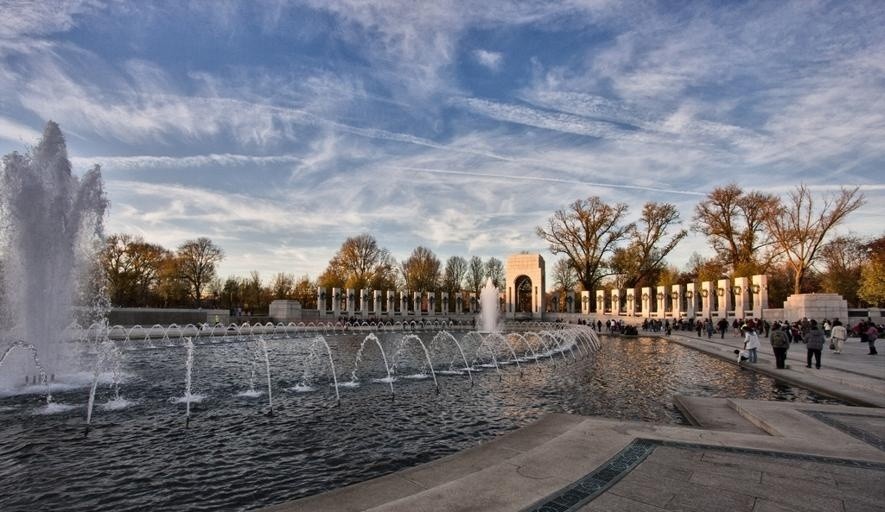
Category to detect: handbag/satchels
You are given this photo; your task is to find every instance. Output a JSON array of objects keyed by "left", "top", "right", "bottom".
[{"left": 829, "top": 343, "right": 834, "bottom": 350}]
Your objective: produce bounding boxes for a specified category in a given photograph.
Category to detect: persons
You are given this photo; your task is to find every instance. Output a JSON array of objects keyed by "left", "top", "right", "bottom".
[
  {"left": 739, "top": 324, "right": 761, "bottom": 363},
  {"left": 732, "top": 316, "right": 842, "bottom": 342},
  {"left": 337, "top": 315, "right": 426, "bottom": 330},
  {"left": 803, "top": 326, "right": 825, "bottom": 370},
  {"left": 830, "top": 320, "right": 848, "bottom": 355},
  {"left": 845, "top": 317, "right": 885, "bottom": 339},
  {"left": 642, "top": 316, "right": 729, "bottom": 339},
  {"left": 734, "top": 348, "right": 751, "bottom": 367},
  {"left": 769, "top": 321, "right": 790, "bottom": 369},
  {"left": 578, "top": 318, "right": 638, "bottom": 335},
  {"left": 780, "top": 323, "right": 794, "bottom": 361},
  {"left": 861, "top": 321, "right": 878, "bottom": 356}
]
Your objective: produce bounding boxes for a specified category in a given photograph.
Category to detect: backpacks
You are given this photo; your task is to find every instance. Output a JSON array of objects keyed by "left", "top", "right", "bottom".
[{"left": 773, "top": 331, "right": 785, "bottom": 345}]
